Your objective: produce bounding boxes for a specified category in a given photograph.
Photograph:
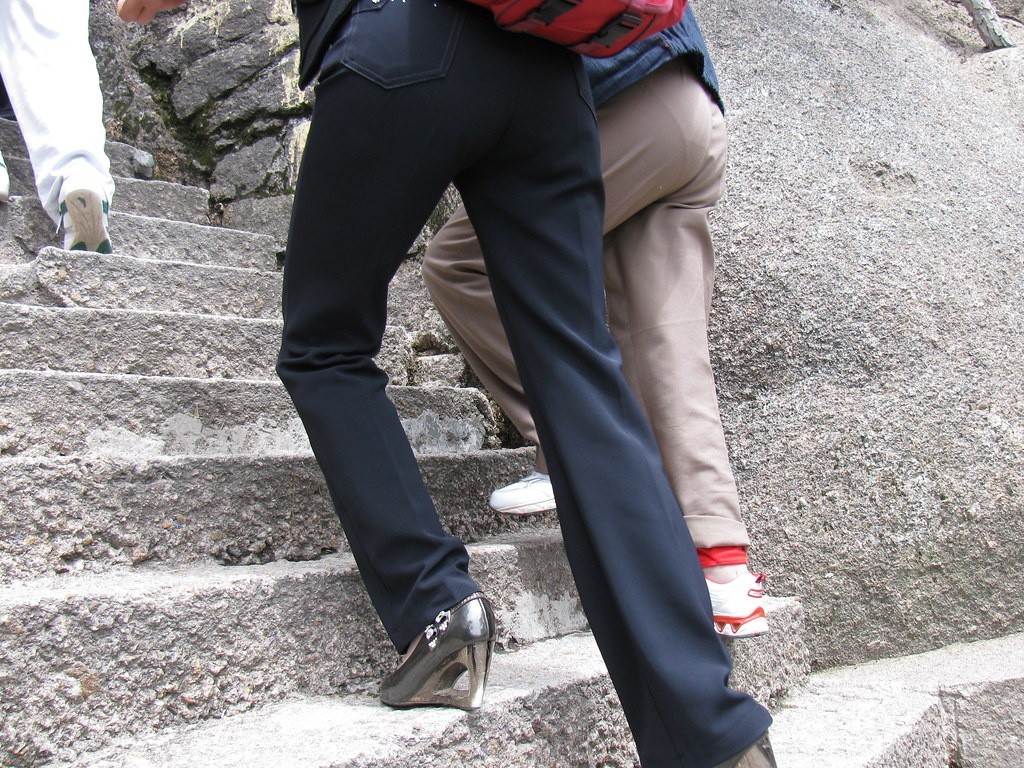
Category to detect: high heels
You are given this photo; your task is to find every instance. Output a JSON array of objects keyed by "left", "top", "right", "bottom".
[
  {"left": 381, "top": 592, "right": 497, "bottom": 709},
  {"left": 716, "top": 729, "right": 778, "bottom": 767}
]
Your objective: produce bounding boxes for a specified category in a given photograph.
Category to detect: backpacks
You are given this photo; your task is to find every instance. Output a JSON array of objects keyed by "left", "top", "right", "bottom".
[{"left": 476, "top": 0, "right": 686, "bottom": 59}]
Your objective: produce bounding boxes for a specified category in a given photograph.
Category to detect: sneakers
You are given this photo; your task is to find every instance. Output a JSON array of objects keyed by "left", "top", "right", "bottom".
[
  {"left": 488, "top": 472, "right": 556, "bottom": 516},
  {"left": 55, "top": 176, "right": 115, "bottom": 254},
  {"left": 704, "top": 571, "right": 768, "bottom": 638}
]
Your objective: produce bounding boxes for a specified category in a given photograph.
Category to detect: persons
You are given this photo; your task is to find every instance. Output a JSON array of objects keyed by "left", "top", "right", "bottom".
[
  {"left": 117, "top": 1, "right": 793, "bottom": 766},
  {"left": 421, "top": 0, "right": 770, "bottom": 638},
  {"left": 0, "top": 0, "right": 117, "bottom": 253}
]
